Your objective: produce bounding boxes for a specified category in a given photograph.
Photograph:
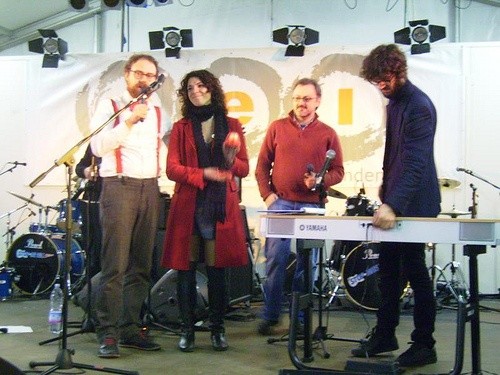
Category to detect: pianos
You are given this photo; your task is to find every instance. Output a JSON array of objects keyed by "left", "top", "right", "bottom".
[{"left": 258, "top": 212, "right": 500, "bottom": 374}]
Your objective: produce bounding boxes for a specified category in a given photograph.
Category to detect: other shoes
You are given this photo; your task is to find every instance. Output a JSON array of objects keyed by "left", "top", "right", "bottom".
[
  {"left": 295, "top": 319, "right": 305, "bottom": 332},
  {"left": 118, "top": 330, "right": 161, "bottom": 351},
  {"left": 179, "top": 332, "right": 195, "bottom": 349},
  {"left": 97, "top": 336, "right": 121, "bottom": 357},
  {"left": 211, "top": 331, "right": 229, "bottom": 351},
  {"left": 351, "top": 325, "right": 398, "bottom": 357},
  {"left": 395, "top": 341, "right": 437, "bottom": 366},
  {"left": 258, "top": 319, "right": 279, "bottom": 327}
]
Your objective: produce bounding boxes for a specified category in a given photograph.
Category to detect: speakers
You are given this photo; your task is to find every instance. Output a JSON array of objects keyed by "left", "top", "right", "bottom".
[{"left": 150, "top": 267, "right": 206, "bottom": 324}]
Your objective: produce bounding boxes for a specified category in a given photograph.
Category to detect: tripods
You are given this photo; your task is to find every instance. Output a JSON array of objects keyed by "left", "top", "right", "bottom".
[
  {"left": 28, "top": 74, "right": 181, "bottom": 375},
  {"left": 267, "top": 169, "right": 368, "bottom": 359},
  {"left": 427, "top": 215, "right": 470, "bottom": 304}
]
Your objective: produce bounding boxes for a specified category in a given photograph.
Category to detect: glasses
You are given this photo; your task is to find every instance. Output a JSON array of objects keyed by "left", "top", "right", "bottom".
[
  {"left": 370, "top": 73, "right": 396, "bottom": 85},
  {"left": 293, "top": 96, "right": 315, "bottom": 103},
  {"left": 129, "top": 69, "right": 156, "bottom": 81}
]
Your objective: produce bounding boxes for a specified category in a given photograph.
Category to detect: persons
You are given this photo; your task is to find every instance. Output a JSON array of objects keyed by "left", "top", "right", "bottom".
[
  {"left": 255, "top": 78, "right": 345, "bottom": 334},
  {"left": 75, "top": 55, "right": 172, "bottom": 357},
  {"left": 163, "top": 71, "right": 250, "bottom": 352},
  {"left": 359, "top": 45, "right": 443, "bottom": 367}
]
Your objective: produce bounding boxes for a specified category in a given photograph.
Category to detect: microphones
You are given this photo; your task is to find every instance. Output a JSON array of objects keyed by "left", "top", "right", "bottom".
[
  {"left": 456, "top": 168, "right": 466, "bottom": 171},
  {"left": 28, "top": 208, "right": 36, "bottom": 216},
  {"left": 140, "top": 93, "right": 147, "bottom": 122},
  {"left": 307, "top": 164, "right": 314, "bottom": 174},
  {"left": 316, "top": 149, "right": 336, "bottom": 185},
  {"left": 8, "top": 162, "right": 27, "bottom": 166}
]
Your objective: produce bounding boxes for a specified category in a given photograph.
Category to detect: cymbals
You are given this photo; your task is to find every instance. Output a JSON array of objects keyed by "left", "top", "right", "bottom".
[
  {"left": 439, "top": 210, "right": 472, "bottom": 215},
  {"left": 7, "top": 191, "right": 45, "bottom": 209},
  {"left": 438, "top": 178, "right": 462, "bottom": 191},
  {"left": 326, "top": 188, "right": 348, "bottom": 199}
]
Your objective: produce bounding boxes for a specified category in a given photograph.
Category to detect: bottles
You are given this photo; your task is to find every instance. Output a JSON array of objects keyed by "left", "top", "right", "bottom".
[{"left": 48, "top": 283, "right": 64, "bottom": 334}]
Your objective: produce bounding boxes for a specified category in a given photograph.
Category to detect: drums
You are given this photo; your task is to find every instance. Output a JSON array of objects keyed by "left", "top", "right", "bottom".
[
  {"left": 57, "top": 198, "right": 83, "bottom": 234},
  {"left": 328, "top": 240, "right": 366, "bottom": 277},
  {"left": 29, "top": 222, "right": 58, "bottom": 234},
  {"left": 0, "top": 265, "right": 12, "bottom": 301},
  {"left": 4, "top": 232, "right": 86, "bottom": 296},
  {"left": 345, "top": 196, "right": 377, "bottom": 216},
  {"left": 341, "top": 241, "right": 411, "bottom": 311}
]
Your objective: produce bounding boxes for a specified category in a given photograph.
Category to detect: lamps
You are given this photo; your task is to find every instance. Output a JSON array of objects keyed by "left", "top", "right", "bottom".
[
  {"left": 28, "top": 29, "right": 68, "bottom": 68},
  {"left": 67, "top": 0, "right": 173, "bottom": 12},
  {"left": 149, "top": 27, "right": 193, "bottom": 59},
  {"left": 273, "top": 25, "right": 319, "bottom": 56},
  {"left": 394, "top": 20, "right": 446, "bottom": 55}
]
